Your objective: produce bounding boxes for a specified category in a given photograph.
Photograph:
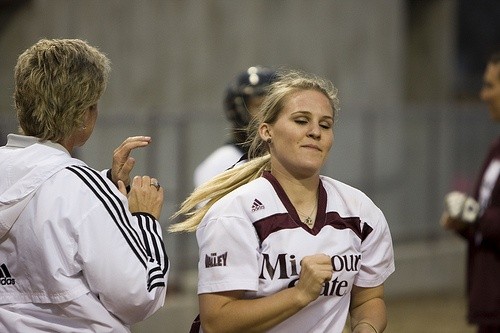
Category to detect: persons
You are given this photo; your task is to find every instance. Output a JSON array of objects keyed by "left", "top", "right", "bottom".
[
  {"left": 437, "top": 53, "right": 500, "bottom": 333},
  {"left": 191, "top": 66, "right": 285, "bottom": 211},
  {"left": 167, "top": 67, "right": 396, "bottom": 333},
  {"left": 0, "top": 38, "right": 172, "bottom": 333}
]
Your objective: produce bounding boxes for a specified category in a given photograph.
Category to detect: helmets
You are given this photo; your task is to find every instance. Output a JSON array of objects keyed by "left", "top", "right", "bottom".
[{"left": 231, "top": 64, "right": 281, "bottom": 97}]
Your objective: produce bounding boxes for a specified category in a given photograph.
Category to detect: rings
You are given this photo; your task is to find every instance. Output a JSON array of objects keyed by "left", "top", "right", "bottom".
[{"left": 150, "top": 181, "right": 161, "bottom": 191}]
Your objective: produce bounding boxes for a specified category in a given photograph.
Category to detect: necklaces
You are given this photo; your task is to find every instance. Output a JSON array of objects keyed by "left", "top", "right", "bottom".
[{"left": 296, "top": 199, "right": 317, "bottom": 226}]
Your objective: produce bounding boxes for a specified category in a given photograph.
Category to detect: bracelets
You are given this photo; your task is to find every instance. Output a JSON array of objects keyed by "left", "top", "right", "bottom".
[{"left": 352, "top": 321, "right": 378, "bottom": 333}]
